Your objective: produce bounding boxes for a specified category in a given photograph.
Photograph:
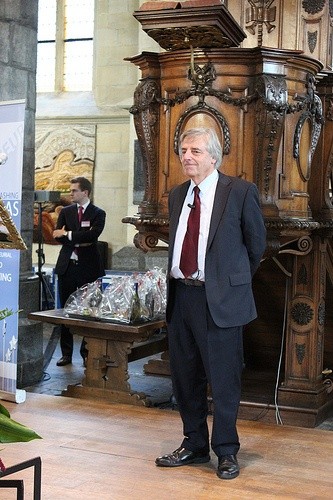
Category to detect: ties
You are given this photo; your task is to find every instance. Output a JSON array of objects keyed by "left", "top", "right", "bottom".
[
  {"left": 78, "top": 206, "right": 83, "bottom": 223},
  {"left": 179, "top": 186, "right": 201, "bottom": 279}
]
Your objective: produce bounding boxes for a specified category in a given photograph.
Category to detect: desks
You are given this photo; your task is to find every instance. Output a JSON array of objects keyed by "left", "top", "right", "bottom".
[{"left": 28, "top": 310, "right": 166, "bottom": 411}]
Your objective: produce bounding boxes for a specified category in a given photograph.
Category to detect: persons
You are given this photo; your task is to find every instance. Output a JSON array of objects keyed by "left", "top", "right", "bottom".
[
  {"left": 154, "top": 128, "right": 266, "bottom": 479},
  {"left": 53, "top": 177, "right": 106, "bottom": 366}
]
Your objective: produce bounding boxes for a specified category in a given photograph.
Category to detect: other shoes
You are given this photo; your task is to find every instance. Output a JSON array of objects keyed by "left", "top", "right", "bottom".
[
  {"left": 83, "top": 359, "right": 88, "bottom": 367},
  {"left": 56, "top": 357, "right": 72, "bottom": 366}
]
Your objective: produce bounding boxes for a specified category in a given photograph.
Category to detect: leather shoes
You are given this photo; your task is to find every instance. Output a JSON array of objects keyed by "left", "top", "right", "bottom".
[
  {"left": 155, "top": 447, "right": 210, "bottom": 467},
  {"left": 217, "top": 454, "right": 239, "bottom": 479}
]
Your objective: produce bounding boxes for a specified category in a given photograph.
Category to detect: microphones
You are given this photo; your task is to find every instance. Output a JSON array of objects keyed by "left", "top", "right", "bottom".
[{"left": 188, "top": 204, "right": 196, "bottom": 208}]
